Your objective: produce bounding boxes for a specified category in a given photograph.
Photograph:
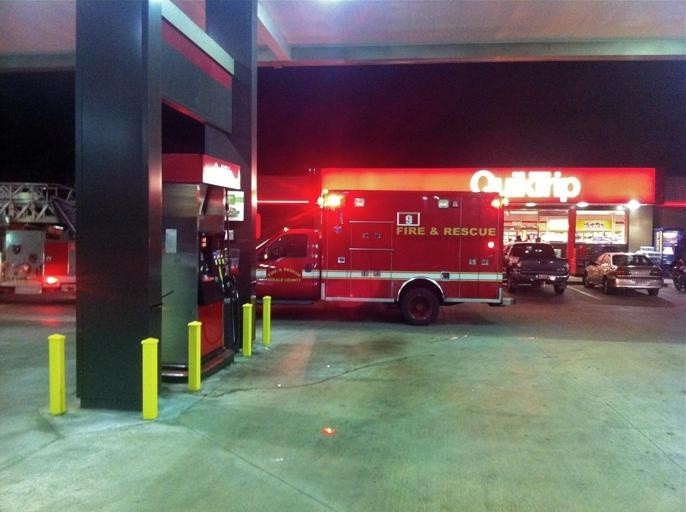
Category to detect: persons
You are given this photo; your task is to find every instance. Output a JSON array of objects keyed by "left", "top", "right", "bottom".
[{"left": 506, "top": 232, "right": 548, "bottom": 245}]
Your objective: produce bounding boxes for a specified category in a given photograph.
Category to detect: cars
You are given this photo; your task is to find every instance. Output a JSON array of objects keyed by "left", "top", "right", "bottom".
[
  {"left": 583, "top": 252, "right": 662, "bottom": 297},
  {"left": 672, "top": 254, "right": 686, "bottom": 292}
]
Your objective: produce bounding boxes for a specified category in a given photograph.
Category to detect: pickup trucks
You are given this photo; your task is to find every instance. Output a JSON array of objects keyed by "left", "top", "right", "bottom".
[{"left": 502, "top": 238, "right": 569, "bottom": 297}]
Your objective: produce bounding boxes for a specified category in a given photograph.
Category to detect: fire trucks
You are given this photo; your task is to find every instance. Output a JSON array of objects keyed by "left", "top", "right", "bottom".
[
  {"left": 0, "top": 182, "right": 78, "bottom": 306},
  {"left": 253, "top": 186, "right": 506, "bottom": 327}
]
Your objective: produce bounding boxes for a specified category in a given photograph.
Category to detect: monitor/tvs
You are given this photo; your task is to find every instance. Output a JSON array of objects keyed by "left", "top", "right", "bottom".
[{"left": 223, "top": 190, "right": 246, "bottom": 222}]
